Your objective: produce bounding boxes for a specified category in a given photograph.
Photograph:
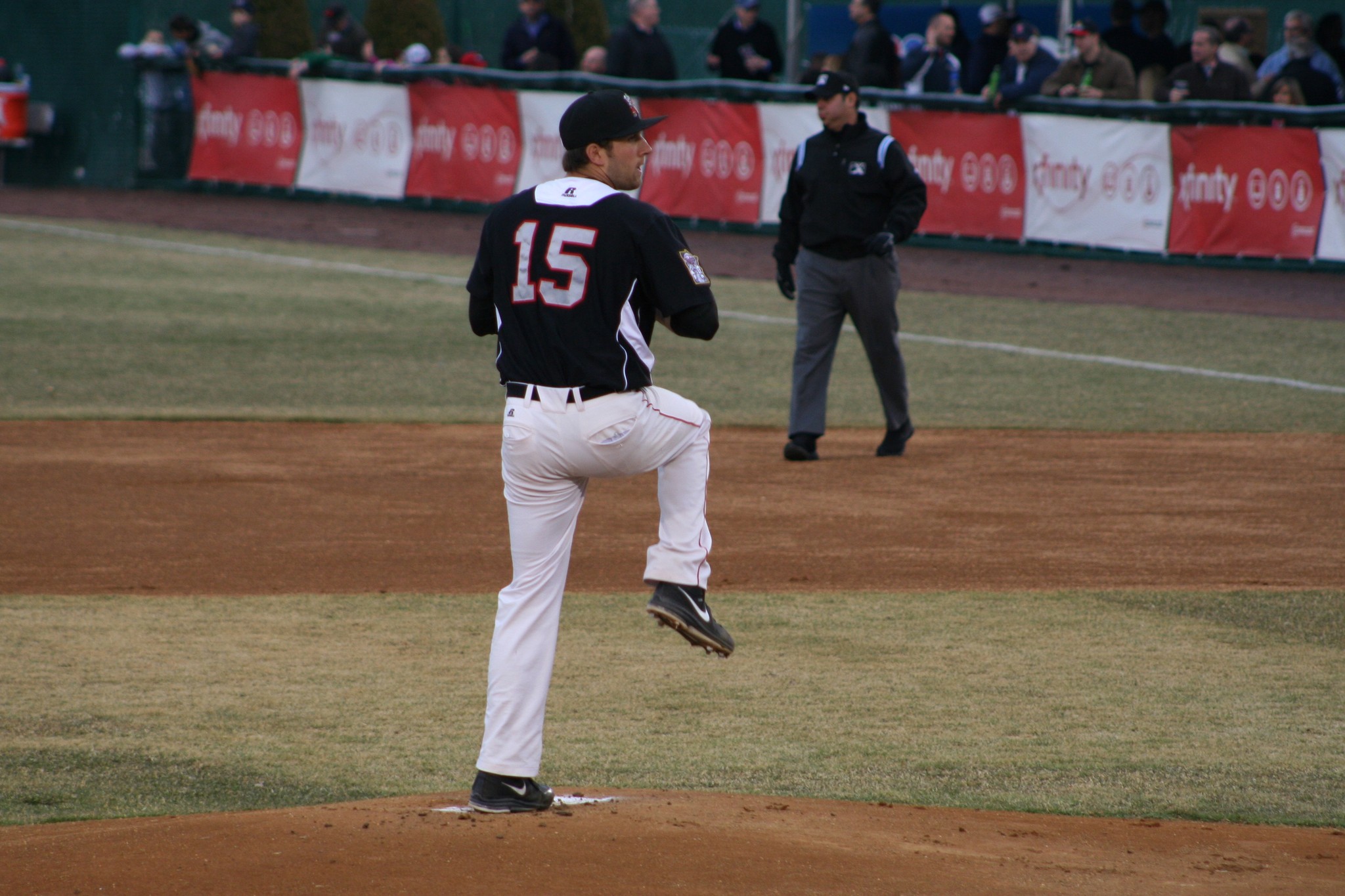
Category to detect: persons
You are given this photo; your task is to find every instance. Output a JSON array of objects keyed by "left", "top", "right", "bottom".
[
  {"left": 769, "top": 70, "right": 930, "bottom": 462},
  {"left": 119, "top": 2, "right": 1345, "bottom": 191},
  {"left": 466, "top": 90, "right": 735, "bottom": 816}
]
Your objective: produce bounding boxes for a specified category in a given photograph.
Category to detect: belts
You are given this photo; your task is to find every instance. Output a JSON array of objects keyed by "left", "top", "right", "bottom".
[{"left": 506, "top": 379, "right": 614, "bottom": 404}]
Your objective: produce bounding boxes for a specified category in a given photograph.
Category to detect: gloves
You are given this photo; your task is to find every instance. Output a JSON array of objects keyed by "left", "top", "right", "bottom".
[
  {"left": 776, "top": 262, "right": 796, "bottom": 299},
  {"left": 873, "top": 231, "right": 895, "bottom": 256}
]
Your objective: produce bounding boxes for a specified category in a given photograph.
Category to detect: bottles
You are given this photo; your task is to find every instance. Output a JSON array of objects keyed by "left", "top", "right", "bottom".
[
  {"left": 1079, "top": 64, "right": 1093, "bottom": 87},
  {"left": 989, "top": 63, "right": 1000, "bottom": 97}
]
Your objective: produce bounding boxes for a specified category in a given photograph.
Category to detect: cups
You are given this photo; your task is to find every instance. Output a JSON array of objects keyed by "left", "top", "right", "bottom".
[{"left": 1174, "top": 80, "right": 1190, "bottom": 101}]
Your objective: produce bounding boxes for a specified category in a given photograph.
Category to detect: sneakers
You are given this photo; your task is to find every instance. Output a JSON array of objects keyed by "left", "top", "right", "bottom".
[
  {"left": 646, "top": 582, "right": 734, "bottom": 657},
  {"left": 468, "top": 769, "right": 554, "bottom": 813}
]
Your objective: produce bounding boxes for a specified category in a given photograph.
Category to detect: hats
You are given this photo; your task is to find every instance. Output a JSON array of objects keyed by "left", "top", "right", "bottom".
[
  {"left": 1007, "top": 19, "right": 1037, "bottom": 43},
  {"left": 462, "top": 53, "right": 488, "bottom": 65},
  {"left": 326, "top": 4, "right": 343, "bottom": 28},
  {"left": 803, "top": 70, "right": 856, "bottom": 103},
  {"left": 978, "top": 2, "right": 1000, "bottom": 24},
  {"left": 740, "top": 0, "right": 758, "bottom": 10},
  {"left": 558, "top": 90, "right": 667, "bottom": 149},
  {"left": 1065, "top": 19, "right": 1098, "bottom": 36}
]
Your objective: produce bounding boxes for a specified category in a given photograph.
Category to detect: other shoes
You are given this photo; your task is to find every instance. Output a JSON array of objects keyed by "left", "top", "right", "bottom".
[
  {"left": 784, "top": 436, "right": 818, "bottom": 461},
  {"left": 877, "top": 419, "right": 914, "bottom": 457}
]
